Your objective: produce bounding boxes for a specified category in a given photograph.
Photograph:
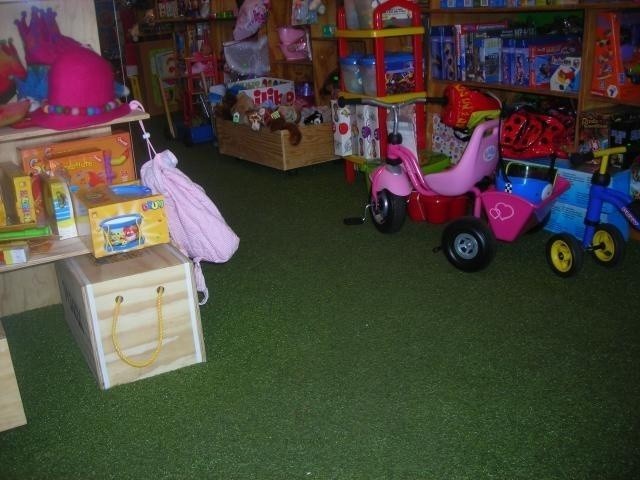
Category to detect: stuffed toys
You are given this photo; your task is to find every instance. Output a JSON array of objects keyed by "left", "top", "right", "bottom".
[{"left": 214, "top": 93, "right": 333, "bottom": 145}]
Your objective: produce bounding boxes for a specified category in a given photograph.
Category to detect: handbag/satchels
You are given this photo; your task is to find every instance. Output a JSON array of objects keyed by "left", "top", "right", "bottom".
[{"left": 140, "top": 149, "right": 240, "bottom": 263}]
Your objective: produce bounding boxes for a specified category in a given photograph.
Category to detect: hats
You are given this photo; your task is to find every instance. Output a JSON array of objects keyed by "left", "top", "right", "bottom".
[{"left": 32, "top": 47, "right": 130, "bottom": 130}]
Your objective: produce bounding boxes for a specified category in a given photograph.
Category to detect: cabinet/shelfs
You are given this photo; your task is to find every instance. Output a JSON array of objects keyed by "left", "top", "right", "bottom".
[{"left": 0, "top": 113, "right": 152, "bottom": 319}]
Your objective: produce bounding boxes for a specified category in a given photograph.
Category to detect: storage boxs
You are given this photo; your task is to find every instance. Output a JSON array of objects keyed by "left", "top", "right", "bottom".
[{"left": 55, "top": 242, "right": 208, "bottom": 390}]
